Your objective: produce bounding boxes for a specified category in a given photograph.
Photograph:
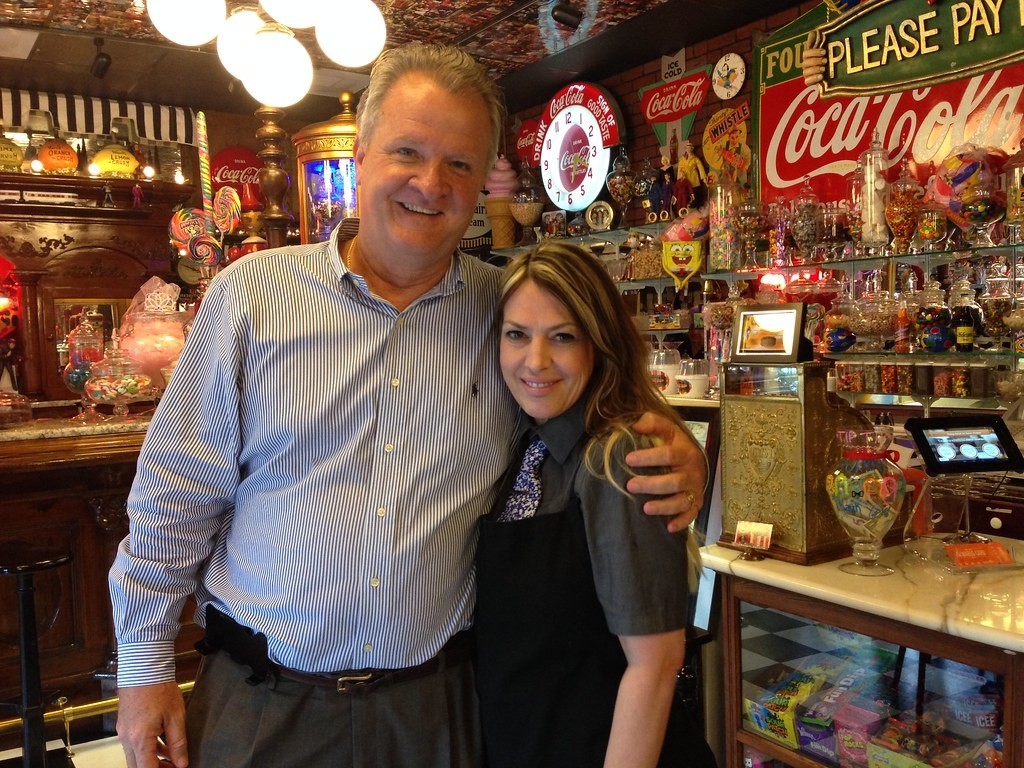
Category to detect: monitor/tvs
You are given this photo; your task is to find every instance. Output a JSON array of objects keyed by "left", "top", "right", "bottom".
[{"left": 906, "top": 412, "right": 1024, "bottom": 477}]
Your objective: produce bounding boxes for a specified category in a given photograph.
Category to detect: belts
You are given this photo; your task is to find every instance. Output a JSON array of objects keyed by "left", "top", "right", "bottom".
[{"left": 263, "top": 643, "right": 451, "bottom": 696}]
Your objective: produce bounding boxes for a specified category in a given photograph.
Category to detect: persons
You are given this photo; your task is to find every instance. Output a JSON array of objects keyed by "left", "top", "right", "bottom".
[
  {"left": 476, "top": 240, "right": 710, "bottom": 768},
  {"left": 645, "top": 138, "right": 711, "bottom": 224},
  {"left": 106, "top": 41, "right": 712, "bottom": 767}
]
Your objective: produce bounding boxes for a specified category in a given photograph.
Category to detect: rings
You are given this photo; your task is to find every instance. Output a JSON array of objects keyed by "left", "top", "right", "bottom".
[{"left": 680, "top": 491, "right": 695, "bottom": 506}]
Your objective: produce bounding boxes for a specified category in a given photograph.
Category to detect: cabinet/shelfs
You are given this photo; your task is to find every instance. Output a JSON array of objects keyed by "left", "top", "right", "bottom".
[
  {"left": 0, "top": 170, "right": 221, "bottom": 744},
  {"left": 695, "top": 526, "right": 1024, "bottom": 768},
  {"left": 701, "top": 241, "right": 1024, "bottom": 472},
  {"left": 488, "top": 216, "right": 721, "bottom": 534}
]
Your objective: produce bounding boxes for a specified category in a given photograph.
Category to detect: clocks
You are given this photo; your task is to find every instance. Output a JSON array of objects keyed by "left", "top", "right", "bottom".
[
  {"left": 530, "top": 82, "right": 628, "bottom": 213},
  {"left": 712, "top": 53, "right": 747, "bottom": 101}
]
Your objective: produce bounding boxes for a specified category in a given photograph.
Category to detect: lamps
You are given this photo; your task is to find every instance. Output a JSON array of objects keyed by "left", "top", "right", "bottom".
[
  {"left": 549, "top": 1, "right": 584, "bottom": 31},
  {"left": 90, "top": 37, "right": 113, "bottom": 80},
  {"left": 144, "top": 0, "right": 389, "bottom": 109}
]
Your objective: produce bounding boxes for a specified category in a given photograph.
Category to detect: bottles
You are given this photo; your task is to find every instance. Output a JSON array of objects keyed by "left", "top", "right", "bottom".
[{"left": 550, "top": 106, "right": 1024, "bottom": 407}]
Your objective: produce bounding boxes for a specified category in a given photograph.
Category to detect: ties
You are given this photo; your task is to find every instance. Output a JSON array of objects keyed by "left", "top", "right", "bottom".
[{"left": 499, "top": 439, "right": 548, "bottom": 521}]
